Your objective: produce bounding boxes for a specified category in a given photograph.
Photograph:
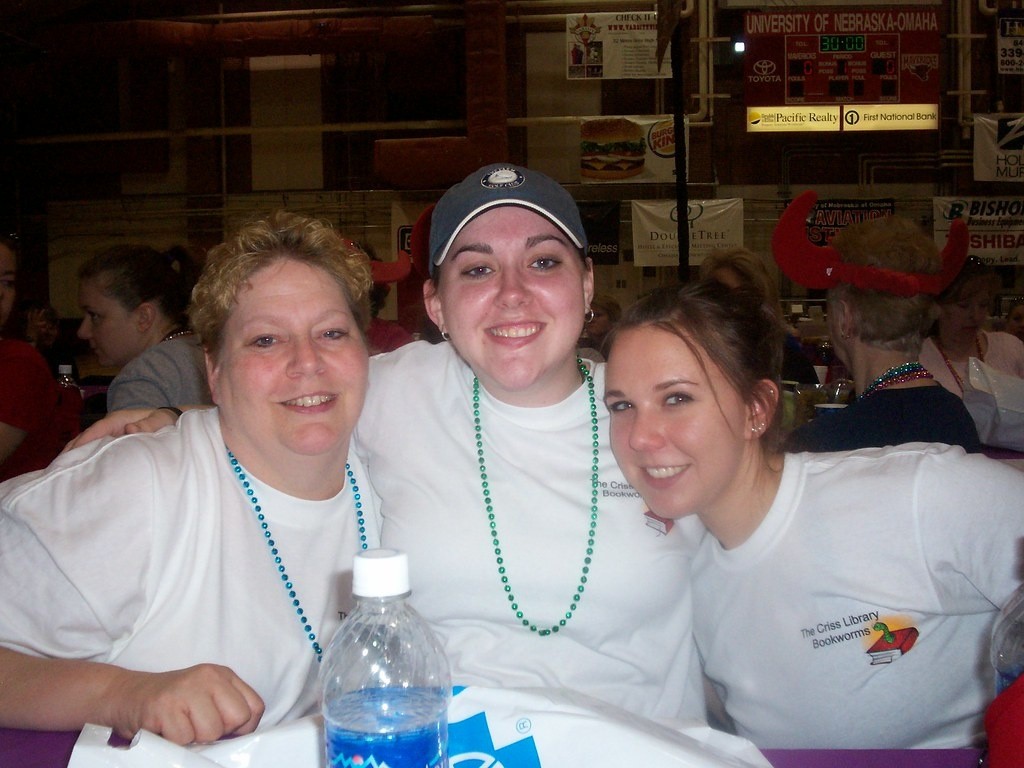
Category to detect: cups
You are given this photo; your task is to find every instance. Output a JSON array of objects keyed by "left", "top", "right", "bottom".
[{"left": 768, "top": 303, "right": 853, "bottom": 434}]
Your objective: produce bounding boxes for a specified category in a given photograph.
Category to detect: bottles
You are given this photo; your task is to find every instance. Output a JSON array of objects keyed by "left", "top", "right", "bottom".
[
  {"left": 319, "top": 548, "right": 449, "bottom": 768},
  {"left": 57, "top": 364, "right": 84, "bottom": 406}
]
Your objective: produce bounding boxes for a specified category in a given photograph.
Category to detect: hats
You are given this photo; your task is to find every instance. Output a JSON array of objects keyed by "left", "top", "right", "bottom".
[{"left": 426, "top": 162, "right": 590, "bottom": 276}]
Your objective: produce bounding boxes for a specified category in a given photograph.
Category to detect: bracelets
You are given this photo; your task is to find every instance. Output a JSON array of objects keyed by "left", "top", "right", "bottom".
[{"left": 159, "top": 407, "right": 183, "bottom": 418}]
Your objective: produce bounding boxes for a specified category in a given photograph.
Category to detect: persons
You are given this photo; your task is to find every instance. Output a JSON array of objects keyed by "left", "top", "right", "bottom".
[
  {"left": 77, "top": 243, "right": 212, "bottom": 415},
  {"left": 0, "top": 236, "right": 60, "bottom": 481},
  {"left": 0, "top": 212, "right": 386, "bottom": 744},
  {"left": 920, "top": 261, "right": 1024, "bottom": 452},
  {"left": 55, "top": 165, "right": 703, "bottom": 732},
  {"left": 365, "top": 285, "right": 414, "bottom": 354},
  {"left": 578, "top": 292, "right": 621, "bottom": 356},
  {"left": 601, "top": 291, "right": 1024, "bottom": 750},
  {"left": 701, "top": 248, "right": 820, "bottom": 393},
  {"left": 785, "top": 214, "right": 981, "bottom": 453}
]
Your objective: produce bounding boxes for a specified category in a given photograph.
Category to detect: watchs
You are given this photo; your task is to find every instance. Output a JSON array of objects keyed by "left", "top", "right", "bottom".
[{"left": 26, "top": 337, "right": 33, "bottom": 342}]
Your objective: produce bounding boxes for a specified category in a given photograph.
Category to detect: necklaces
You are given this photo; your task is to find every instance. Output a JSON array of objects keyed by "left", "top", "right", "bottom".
[
  {"left": 224, "top": 443, "right": 368, "bottom": 663},
  {"left": 473, "top": 357, "right": 600, "bottom": 634},
  {"left": 859, "top": 362, "right": 933, "bottom": 400},
  {"left": 935, "top": 337, "right": 984, "bottom": 393},
  {"left": 164, "top": 330, "right": 192, "bottom": 340}
]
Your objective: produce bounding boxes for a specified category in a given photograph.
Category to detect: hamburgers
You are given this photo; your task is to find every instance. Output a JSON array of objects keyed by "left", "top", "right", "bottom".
[{"left": 579, "top": 117, "right": 647, "bottom": 179}]
